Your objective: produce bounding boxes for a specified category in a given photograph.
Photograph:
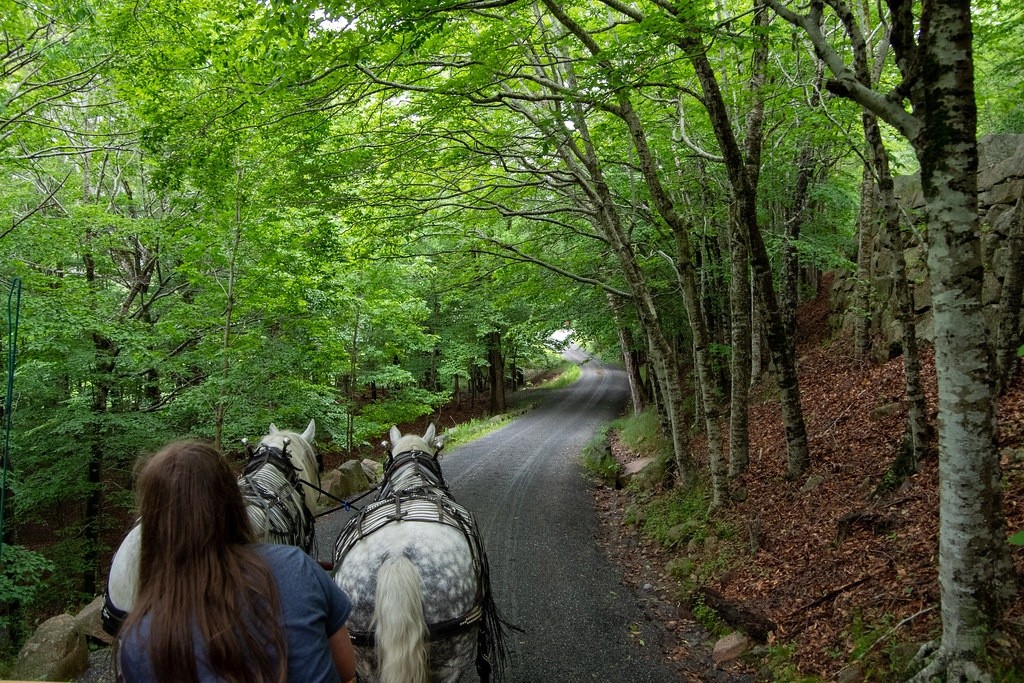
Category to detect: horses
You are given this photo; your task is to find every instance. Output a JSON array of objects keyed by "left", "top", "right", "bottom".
[
  {"left": 106, "top": 417, "right": 321, "bottom": 618},
  {"left": 328, "top": 422, "right": 485, "bottom": 683}
]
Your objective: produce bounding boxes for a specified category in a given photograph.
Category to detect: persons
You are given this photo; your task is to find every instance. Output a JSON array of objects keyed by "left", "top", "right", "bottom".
[{"left": 105, "top": 442, "right": 357, "bottom": 682}]
